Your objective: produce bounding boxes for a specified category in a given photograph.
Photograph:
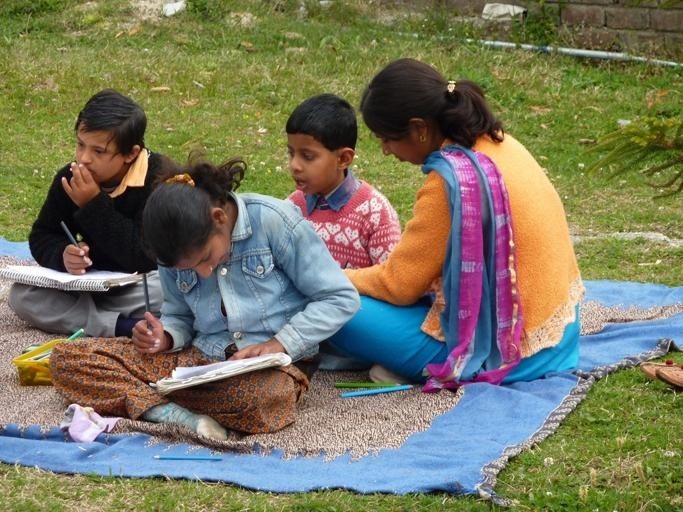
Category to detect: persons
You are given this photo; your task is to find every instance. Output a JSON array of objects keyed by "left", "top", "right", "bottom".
[
  {"left": 5, "top": 90, "right": 179, "bottom": 339},
  {"left": 277, "top": 94, "right": 402, "bottom": 296},
  {"left": 48, "top": 150, "right": 359, "bottom": 444},
  {"left": 321, "top": 60, "right": 584, "bottom": 392}
]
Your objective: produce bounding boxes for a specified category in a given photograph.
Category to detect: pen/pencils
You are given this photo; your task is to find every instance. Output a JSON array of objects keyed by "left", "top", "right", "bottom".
[
  {"left": 333, "top": 382, "right": 414, "bottom": 398},
  {"left": 57, "top": 326, "right": 84, "bottom": 342},
  {"left": 142, "top": 271, "right": 151, "bottom": 332},
  {"left": 154, "top": 456, "right": 224, "bottom": 461},
  {"left": 59, "top": 219, "right": 78, "bottom": 249},
  {"left": 26, "top": 351, "right": 51, "bottom": 361}
]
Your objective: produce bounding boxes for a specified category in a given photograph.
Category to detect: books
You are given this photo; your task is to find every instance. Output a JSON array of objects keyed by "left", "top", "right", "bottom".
[
  {"left": 156, "top": 352, "right": 292, "bottom": 396},
  {"left": 3, "top": 266, "right": 140, "bottom": 293}
]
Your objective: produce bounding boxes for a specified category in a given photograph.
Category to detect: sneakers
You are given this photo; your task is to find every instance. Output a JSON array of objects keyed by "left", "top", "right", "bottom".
[{"left": 638, "top": 361, "right": 683, "bottom": 388}]
